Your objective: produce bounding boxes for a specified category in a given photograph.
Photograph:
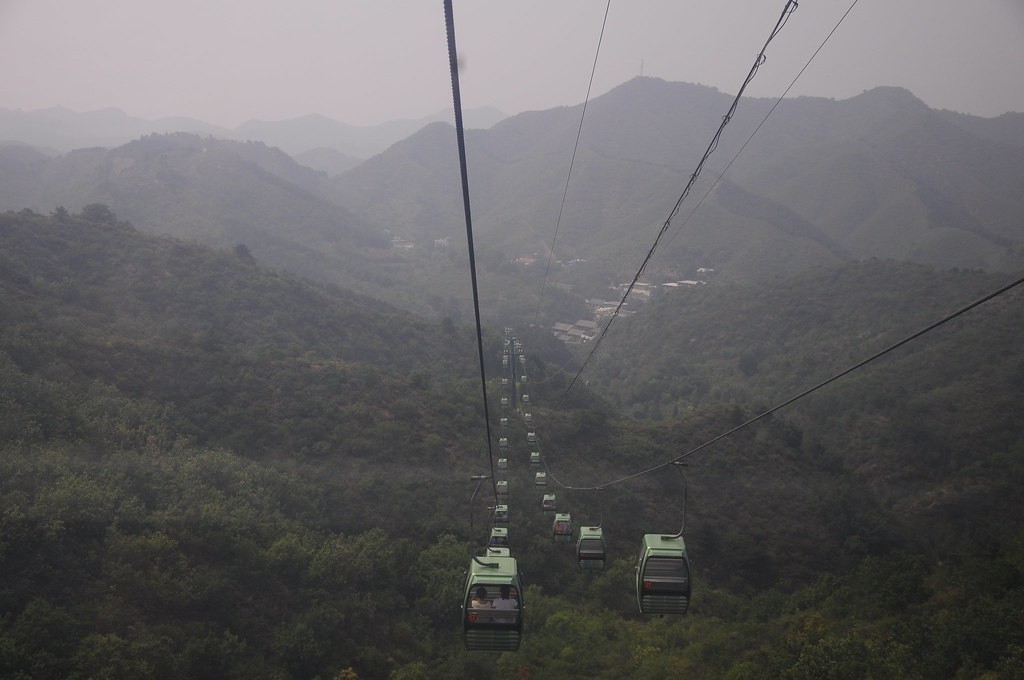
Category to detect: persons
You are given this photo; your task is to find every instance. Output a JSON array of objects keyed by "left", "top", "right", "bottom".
[
  {"left": 492, "top": 537, "right": 498, "bottom": 544},
  {"left": 492, "top": 587, "right": 518, "bottom": 623},
  {"left": 501, "top": 537, "right": 508, "bottom": 545},
  {"left": 544, "top": 502, "right": 549, "bottom": 507},
  {"left": 556, "top": 521, "right": 565, "bottom": 533},
  {"left": 472, "top": 588, "right": 492, "bottom": 623}
]
